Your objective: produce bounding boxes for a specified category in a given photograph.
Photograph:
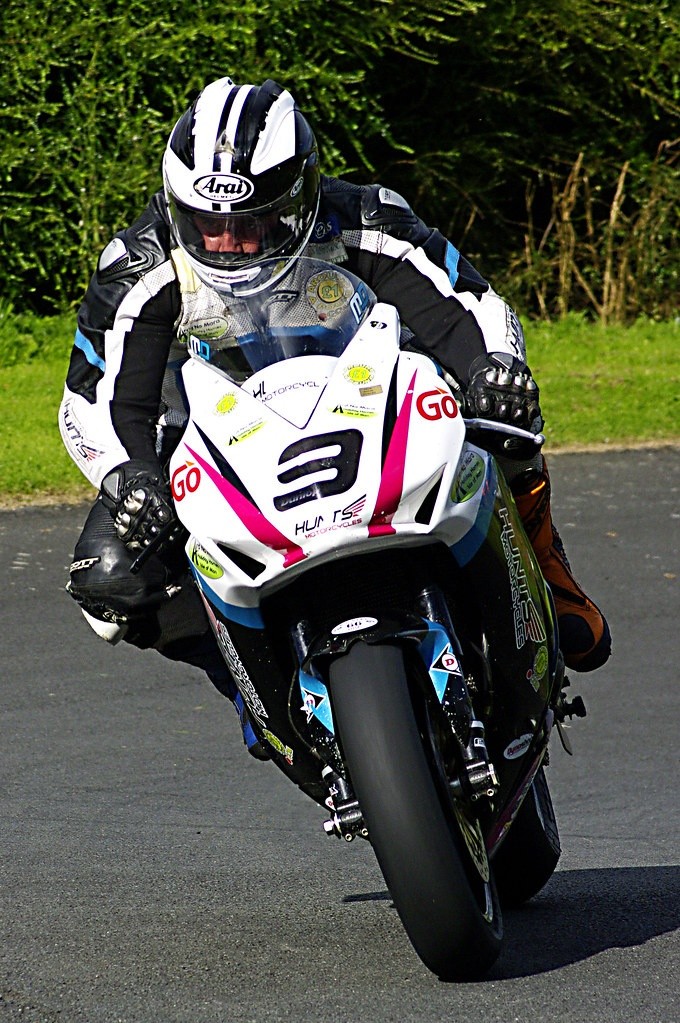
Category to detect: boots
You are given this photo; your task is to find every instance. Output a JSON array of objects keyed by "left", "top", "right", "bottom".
[
  {"left": 206, "top": 665, "right": 270, "bottom": 763},
  {"left": 514, "top": 453, "right": 612, "bottom": 673}
]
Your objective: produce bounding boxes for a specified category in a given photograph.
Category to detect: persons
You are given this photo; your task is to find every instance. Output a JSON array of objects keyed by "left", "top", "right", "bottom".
[{"left": 59, "top": 78, "right": 611, "bottom": 819}]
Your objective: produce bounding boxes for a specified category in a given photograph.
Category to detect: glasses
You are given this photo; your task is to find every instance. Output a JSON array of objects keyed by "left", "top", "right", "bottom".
[{"left": 183, "top": 210, "right": 278, "bottom": 244}]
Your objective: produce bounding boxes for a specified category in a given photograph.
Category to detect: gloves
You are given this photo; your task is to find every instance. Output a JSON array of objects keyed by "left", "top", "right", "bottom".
[
  {"left": 97, "top": 459, "right": 184, "bottom": 564},
  {"left": 465, "top": 352, "right": 544, "bottom": 449}
]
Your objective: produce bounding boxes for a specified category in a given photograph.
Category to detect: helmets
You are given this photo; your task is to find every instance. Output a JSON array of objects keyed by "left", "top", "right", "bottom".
[{"left": 162, "top": 77, "right": 320, "bottom": 299}]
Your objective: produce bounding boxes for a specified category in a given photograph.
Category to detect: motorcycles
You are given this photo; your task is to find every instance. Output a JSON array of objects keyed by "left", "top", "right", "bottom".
[{"left": 127, "top": 253, "right": 588, "bottom": 988}]
